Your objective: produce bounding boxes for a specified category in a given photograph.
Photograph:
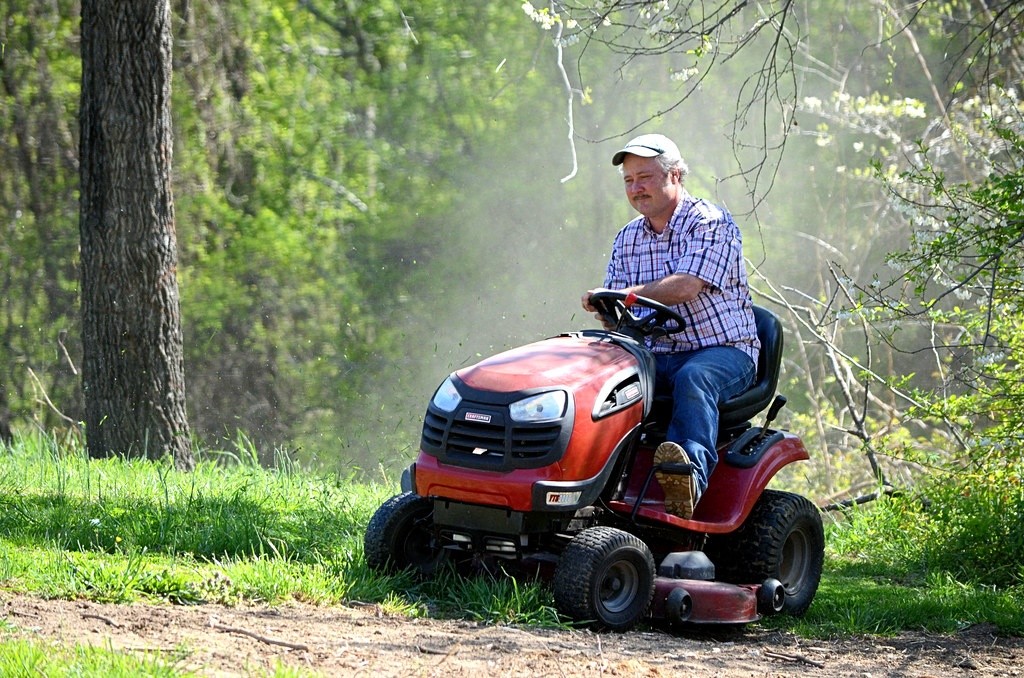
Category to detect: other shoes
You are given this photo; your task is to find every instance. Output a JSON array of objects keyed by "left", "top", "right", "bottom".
[{"left": 654, "top": 442, "right": 696, "bottom": 521}]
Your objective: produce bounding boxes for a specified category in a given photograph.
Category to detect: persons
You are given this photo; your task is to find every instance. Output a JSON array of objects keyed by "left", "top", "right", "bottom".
[{"left": 580, "top": 133, "right": 763, "bottom": 520}]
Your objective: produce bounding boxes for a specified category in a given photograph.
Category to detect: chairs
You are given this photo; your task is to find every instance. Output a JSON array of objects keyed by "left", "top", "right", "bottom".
[{"left": 650, "top": 305, "right": 784, "bottom": 426}]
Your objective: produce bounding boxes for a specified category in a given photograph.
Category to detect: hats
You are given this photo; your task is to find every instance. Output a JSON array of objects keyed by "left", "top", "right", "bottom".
[{"left": 612, "top": 134, "right": 680, "bottom": 167}]
